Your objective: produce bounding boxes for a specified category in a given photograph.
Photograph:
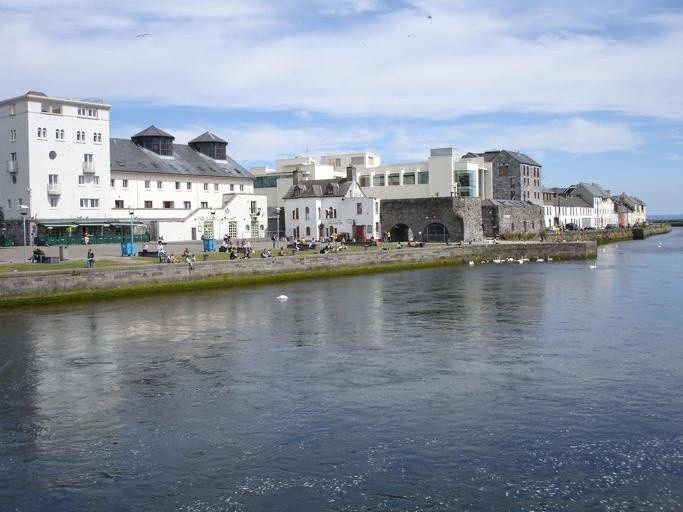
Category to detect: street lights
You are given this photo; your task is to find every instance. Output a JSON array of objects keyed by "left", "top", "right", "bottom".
[
  {"left": 209, "top": 206, "right": 217, "bottom": 253},
  {"left": 325, "top": 208, "right": 331, "bottom": 240},
  {"left": 274, "top": 206, "right": 283, "bottom": 248},
  {"left": 16, "top": 204, "right": 30, "bottom": 264},
  {"left": 127, "top": 205, "right": 137, "bottom": 257}
]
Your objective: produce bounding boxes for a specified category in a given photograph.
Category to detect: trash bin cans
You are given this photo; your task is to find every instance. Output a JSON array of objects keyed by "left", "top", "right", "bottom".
[
  {"left": 203, "top": 239, "right": 217, "bottom": 252},
  {"left": 121, "top": 241, "right": 138, "bottom": 256},
  {"left": 59, "top": 245, "right": 69, "bottom": 261}
]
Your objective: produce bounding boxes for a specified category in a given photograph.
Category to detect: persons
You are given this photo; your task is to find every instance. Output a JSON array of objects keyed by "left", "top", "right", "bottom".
[
  {"left": 157, "top": 240, "right": 195, "bottom": 263},
  {"left": 291, "top": 237, "right": 341, "bottom": 254},
  {"left": 84, "top": 231, "right": 89, "bottom": 244},
  {"left": 189, "top": 263, "right": 193, "bottom": 274},
  {"left": 88, "top": 248, "right": 94, "bottom": 268},
  {"left": 28, "top": 248, "right": 44, "bottom": 263},
  {"left": 493, "top": 237, "right": 498, "bottom": 244},
  {"left": 352, "top": 231, "right": 425, "bottom": 249},
  {"left": 143, "top": 241, "right": 147, "bottom": 256},
  {"left": 223, "top": 233, "right": 287, "bottom": 260}
]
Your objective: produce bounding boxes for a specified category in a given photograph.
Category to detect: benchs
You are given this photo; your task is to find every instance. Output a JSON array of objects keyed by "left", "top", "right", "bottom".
[
  {"left": 344, "top": 242, "right": 367, "bottom": 246},
  {"left": 288, "top": 244, "right": 316, "bottom": 249},
  {"left": 229, "top": 248, "right": 246, "bottom": 253},
  {"left": 138, "top": 252, "right": 158, "bottom": 257},
  {"left": 37, "top": 257, "right": 59, "bottom": 264}
]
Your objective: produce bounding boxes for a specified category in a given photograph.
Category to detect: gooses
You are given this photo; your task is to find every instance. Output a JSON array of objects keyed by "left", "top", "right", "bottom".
[
  {"left": 469, "top": 260, "right": 474, "bottom": 264},
  {"left": 590, "top": 262, "right": 596, "bottom": 269},
  {"left": 276, "top": 295, "right": 289, "bottom": 299},
  {"left": 536, "top": 255, "right": 544, "bottom": 262},
  {"left": 492, "top": 256, "right": 529, "bottom": 264},
  {"left": 548, "top": 256, "right": 553, "bottom": 260}
]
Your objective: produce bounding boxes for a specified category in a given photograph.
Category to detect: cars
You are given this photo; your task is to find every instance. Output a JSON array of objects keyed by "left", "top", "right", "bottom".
[{"left": 547, "top": 221, "right": 654, "bottom": 234}]
